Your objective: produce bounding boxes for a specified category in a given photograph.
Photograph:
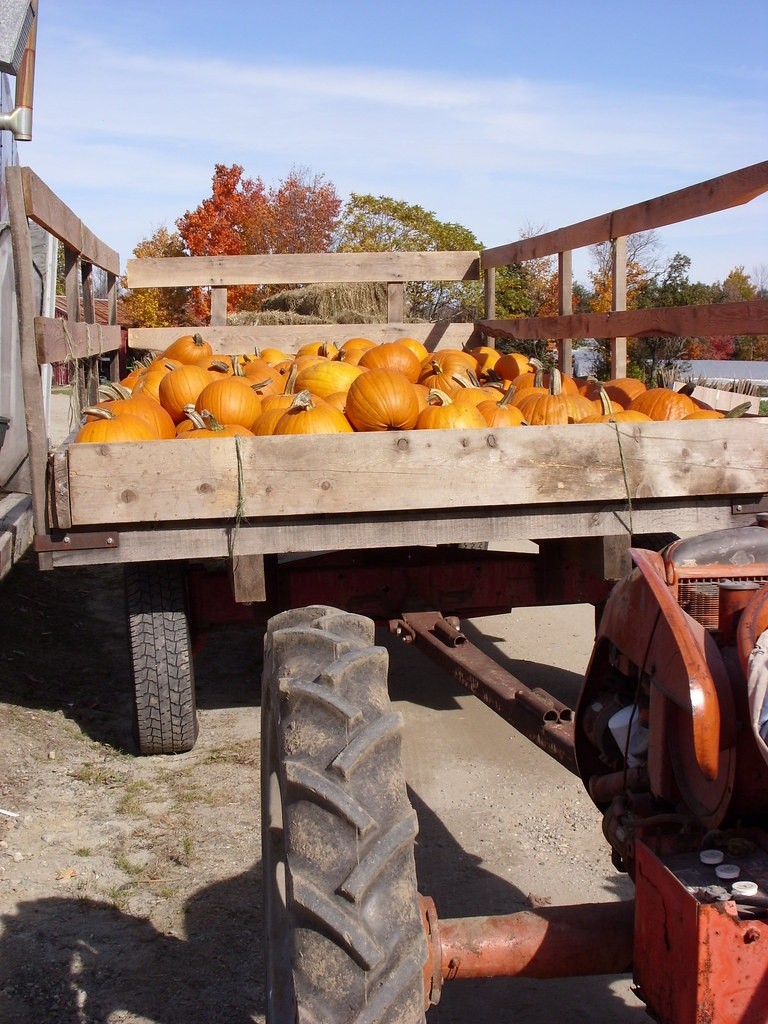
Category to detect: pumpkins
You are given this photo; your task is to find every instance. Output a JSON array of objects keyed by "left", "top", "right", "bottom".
[{"left": 73, "top": 333, "right": 753, "bottom": 443}]
